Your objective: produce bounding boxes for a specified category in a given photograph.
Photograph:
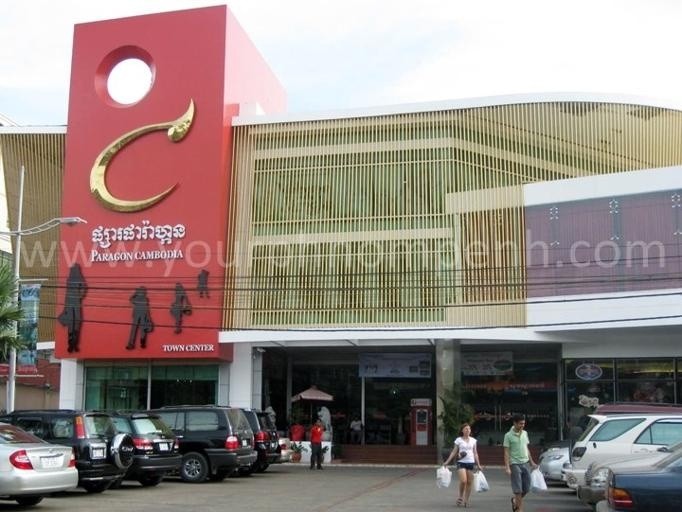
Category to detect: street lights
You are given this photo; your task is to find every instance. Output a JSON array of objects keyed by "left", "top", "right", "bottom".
[{"left": 0, "top": 164, "right": 89, "bottom": 415}]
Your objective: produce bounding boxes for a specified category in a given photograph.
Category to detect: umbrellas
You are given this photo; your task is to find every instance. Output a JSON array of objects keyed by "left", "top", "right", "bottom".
[{"left": 292, "top": 385, "right": 334, "bottom": 424}]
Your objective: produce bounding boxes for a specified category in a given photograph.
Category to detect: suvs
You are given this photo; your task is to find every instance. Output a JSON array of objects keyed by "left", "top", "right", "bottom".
[{"left": 1, "top": 404, "right": 293, "bottom": 506}]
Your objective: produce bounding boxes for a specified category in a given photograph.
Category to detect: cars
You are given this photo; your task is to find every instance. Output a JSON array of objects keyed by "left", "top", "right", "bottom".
[{"left": 536, "top": 401, "right": 681, "bottom": 511}]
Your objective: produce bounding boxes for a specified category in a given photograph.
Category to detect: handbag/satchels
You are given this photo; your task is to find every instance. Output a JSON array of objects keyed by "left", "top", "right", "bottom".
[
  {"left": 436, "top": 466, "right": 453, "bottom": 490},
  {"left": 473, "top": 468, "right": 490, "bottom": 493},
  {"left": 530, "top": 466, "right": 548, "bottom": 495}
]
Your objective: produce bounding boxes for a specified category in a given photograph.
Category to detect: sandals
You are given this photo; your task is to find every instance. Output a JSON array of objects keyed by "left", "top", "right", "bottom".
[
  {"left": 456, "top": 497, "right": 472, "bottom": 508},
  {"left": 511, "top": 497, "right": 518, "bottom": 512}
]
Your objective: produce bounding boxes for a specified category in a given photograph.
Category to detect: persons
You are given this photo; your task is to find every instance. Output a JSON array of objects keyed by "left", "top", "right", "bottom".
[
  {"left": 503, "top": 414, "right": 537, "bottom": 512},
  {"left": 443, "top": 422, "right": 483, "bottom": 508},
  {"left": 318, "top": 406, "right": 362, "bottom": 444},
  {"left": 310, "top": 419, "right": 324, "bottom": 470}
]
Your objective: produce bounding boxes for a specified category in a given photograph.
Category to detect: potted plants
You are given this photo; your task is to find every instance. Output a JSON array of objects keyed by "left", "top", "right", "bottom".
[
  {"left": 290, "top": 441, "right": 307, "bottom": 462},
  {"left": 437, "top": 380, "right": 475, "bottom": 465},
  {"left": 310, "top": 445, "right": 329, "bottom": 463}
]
